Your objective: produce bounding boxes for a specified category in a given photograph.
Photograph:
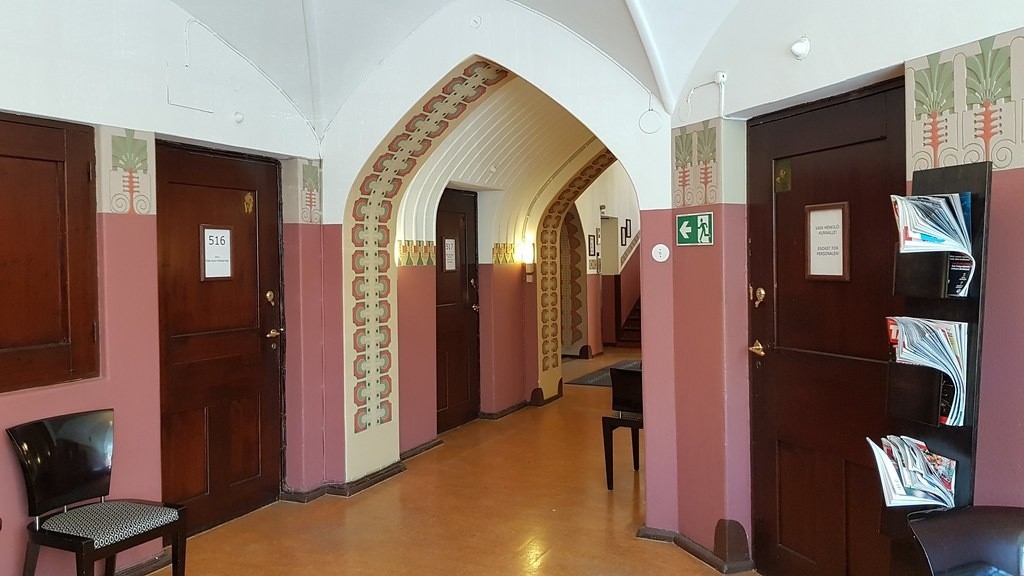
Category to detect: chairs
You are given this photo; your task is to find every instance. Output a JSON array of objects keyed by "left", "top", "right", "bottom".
[
  {"left": 602, "top": 367, "right": 643, "bottom": 491},
  {"left": 5, "top": 407, "right": 190, "bottom": 576}
]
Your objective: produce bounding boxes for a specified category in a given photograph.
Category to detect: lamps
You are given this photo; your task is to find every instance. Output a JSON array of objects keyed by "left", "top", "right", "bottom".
[{"left": 522, "top": 230, "right": 538, "bottom": 284}]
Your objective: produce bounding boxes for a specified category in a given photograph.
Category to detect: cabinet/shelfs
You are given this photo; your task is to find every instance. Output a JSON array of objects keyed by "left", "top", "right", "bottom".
[{"left": 876, "top": 159, "right": 992, "bottom": 575}]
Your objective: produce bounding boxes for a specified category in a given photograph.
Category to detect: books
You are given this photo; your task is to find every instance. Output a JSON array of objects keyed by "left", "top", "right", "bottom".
[
  {"left": 866, "top": 435, "right": 956, "bottom": 508},
  {"left": 887, "top": 317, "right": 968, "bottom": 427},
  {"left": 890, "top": 192, "right": 977, "bottom": 298}
]
